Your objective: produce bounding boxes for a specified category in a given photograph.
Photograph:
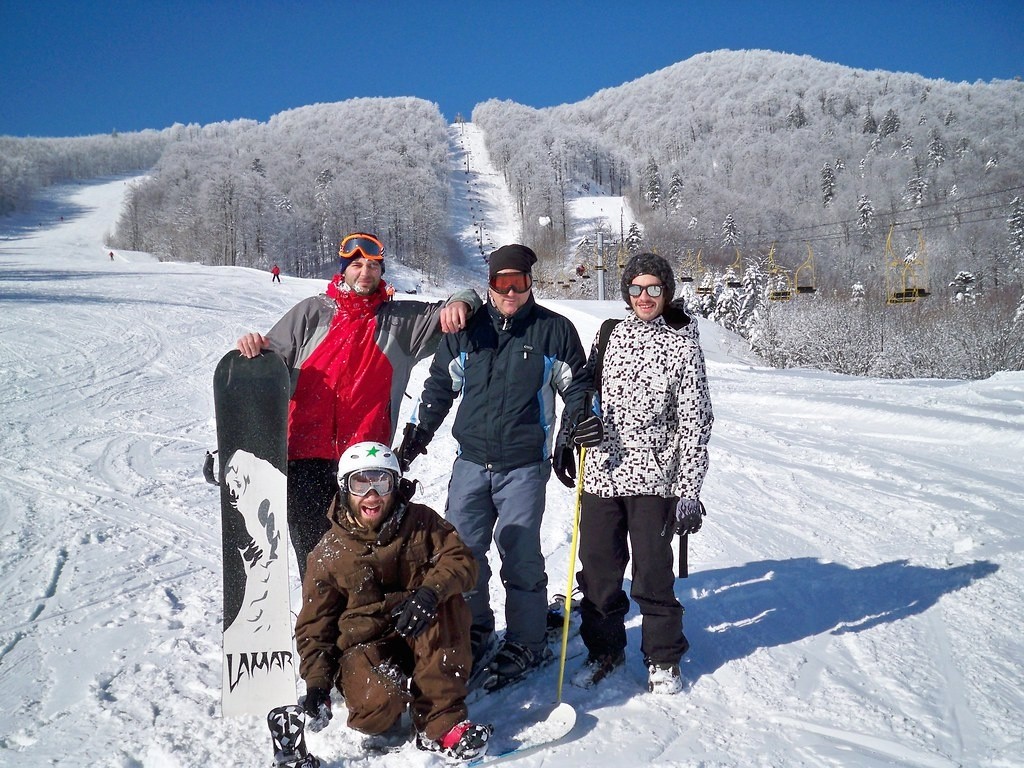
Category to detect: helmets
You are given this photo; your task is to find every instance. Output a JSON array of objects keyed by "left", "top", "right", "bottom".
[{"left": 338, "top": 441, "right": 402, "bottom": 480}]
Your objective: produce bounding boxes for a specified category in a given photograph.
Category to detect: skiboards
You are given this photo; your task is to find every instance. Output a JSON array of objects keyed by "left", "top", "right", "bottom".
[{"left": 361, "top": 594, "right": 587, "bottom": 768}]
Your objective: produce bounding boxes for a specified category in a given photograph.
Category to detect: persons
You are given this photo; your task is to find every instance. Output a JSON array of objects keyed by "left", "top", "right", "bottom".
[
  {"left": 576, "top": 265, "right": 585, "bottom": 276},
  {"left": 236, "top": 232, "right": 483, "bottom": 583},
  {"left": 386, "top": 284, "right": 395, "bottom": 302},
  {"left": 393, "top": 244, "right": 604, "bottom": 679},
  {"left": 108, "top": 251, "right": 114, "bottom": 261},
  {"left": 271, "top": 265, "right": 281, "bottom": 284},
  {"left": 293, "top": 441, "right": 493, "bottom": 763},
  {"left": 552, "top": 253, "right": 714, "bottom": 695}
]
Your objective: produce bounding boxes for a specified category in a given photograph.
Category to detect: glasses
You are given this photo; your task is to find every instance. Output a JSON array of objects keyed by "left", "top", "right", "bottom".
[
  {"left": 338, "top": 234, "right": 385, "bottom": 259},
  {"left": 625, "top": 283, "right": 667, "bottom": 297},
  {"left": 489, "top": 272, "right": 532, "bottom": 294},
  {"left": 348, "top": 468, "right": 394, "bottom": 497}
]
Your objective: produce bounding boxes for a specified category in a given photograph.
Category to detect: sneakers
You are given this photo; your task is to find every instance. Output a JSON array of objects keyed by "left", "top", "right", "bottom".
[
  {"left": 649, "top": 663, "right": 683, "bottom": 695},
  {"left": 490, "top": 640, "right": 542, "bottom": 676},
  {"left": 470, "top": 624, "right": 495, "bottom": 666},
  {"left": 419, "top": 720, "right": 488, "bottom": 749},
  {"left": 572, "top": 648, "right": 626, "bottom": 688}
]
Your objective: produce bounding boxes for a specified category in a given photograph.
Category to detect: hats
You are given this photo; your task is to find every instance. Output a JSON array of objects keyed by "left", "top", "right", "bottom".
[
  {"left": 340, "top": 232, "right": 385, "bottom": 274},
  {"left": 621, "top": 253, "right": 675, "bottom": 307},
  {"left": 489, "top": 244, "right": 538, "bottom": 275}
]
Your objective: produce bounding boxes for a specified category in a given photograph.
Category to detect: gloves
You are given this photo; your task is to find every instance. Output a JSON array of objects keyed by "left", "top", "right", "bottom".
[
  {"left": 667, "top": 496, "right": 702, "bottom": 536},
  {"left": 573, "top": 415, "right": 604, "bottom": 447},
  {"left": 390, "top": 586, "right": 441, "bottom": 639},
  {"left": 303, "top": 687, "right": 331, "bottom": 719},
  {"left": 553, "top": 446, "right": 576, "bottom": 488},
  {"left": 394, "top": 424, "right": 432, "bottom": 478}
]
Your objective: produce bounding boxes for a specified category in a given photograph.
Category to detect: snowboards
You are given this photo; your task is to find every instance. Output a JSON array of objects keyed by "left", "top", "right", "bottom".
[{"left": 212, "top": 349, "right": 299, "bottom": 722}]
